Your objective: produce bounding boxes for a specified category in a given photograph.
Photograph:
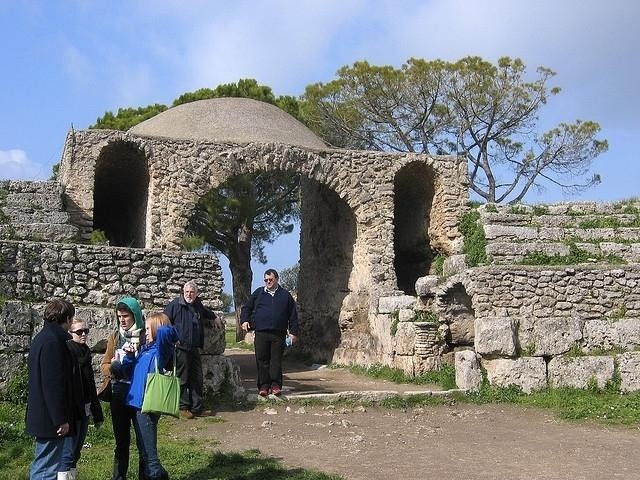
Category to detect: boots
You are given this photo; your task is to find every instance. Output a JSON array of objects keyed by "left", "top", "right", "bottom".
[{"left": 57, "top": 468, "right": 76, "bottom": 480}]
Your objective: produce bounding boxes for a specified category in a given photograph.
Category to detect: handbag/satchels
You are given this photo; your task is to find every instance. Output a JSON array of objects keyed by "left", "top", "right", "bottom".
[
  {"left": 140, "top": 373, "right": 181, "bottom": 419},
  {"left": 96, "top": 378, "right": 111, "bottom": 402},
  {"left": 247, "top": 310, "right": 256, "bottom": 332}
]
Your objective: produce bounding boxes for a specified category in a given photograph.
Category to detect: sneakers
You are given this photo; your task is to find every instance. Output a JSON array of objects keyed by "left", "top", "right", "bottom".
[
  {"left": 194, "top": 408, "right": 213, "bottom": 417},
  {"left": 259, "top": 387, "right": 269, "bottom": 395},
  {"left": 271, "top": 386, "right": 282, "bottom": 395},
  {"left": 179, "top": 409, "right": 194, "bottom": 419}
]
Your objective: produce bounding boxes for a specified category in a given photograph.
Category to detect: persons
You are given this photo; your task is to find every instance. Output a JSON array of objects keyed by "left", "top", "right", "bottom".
[
  {"left": 123, "top": 314, "right": 181, "bottom": 480},
  {"left": 26, "top": 299, "right": 79, "bottom": 480},
  {"left": 163, "top": 280, "right": 225, "bottom": 418},
  {"left": 101, "top": 295, "right": 144, "bottom": 480},
  {"left": 59, "top": 319, "right": 103, "bottom": 479},
  {"left": 239, "top": 269, "right": 299, "bottom": 396}
]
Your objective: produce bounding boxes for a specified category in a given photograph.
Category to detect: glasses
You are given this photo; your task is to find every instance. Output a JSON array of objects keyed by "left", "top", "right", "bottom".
[
  {"left": 264, "top": 278, "right": 275, "bottom": 282},
  {"left": 70, "top": 328, "right": 89, "bottom": 335}
]
